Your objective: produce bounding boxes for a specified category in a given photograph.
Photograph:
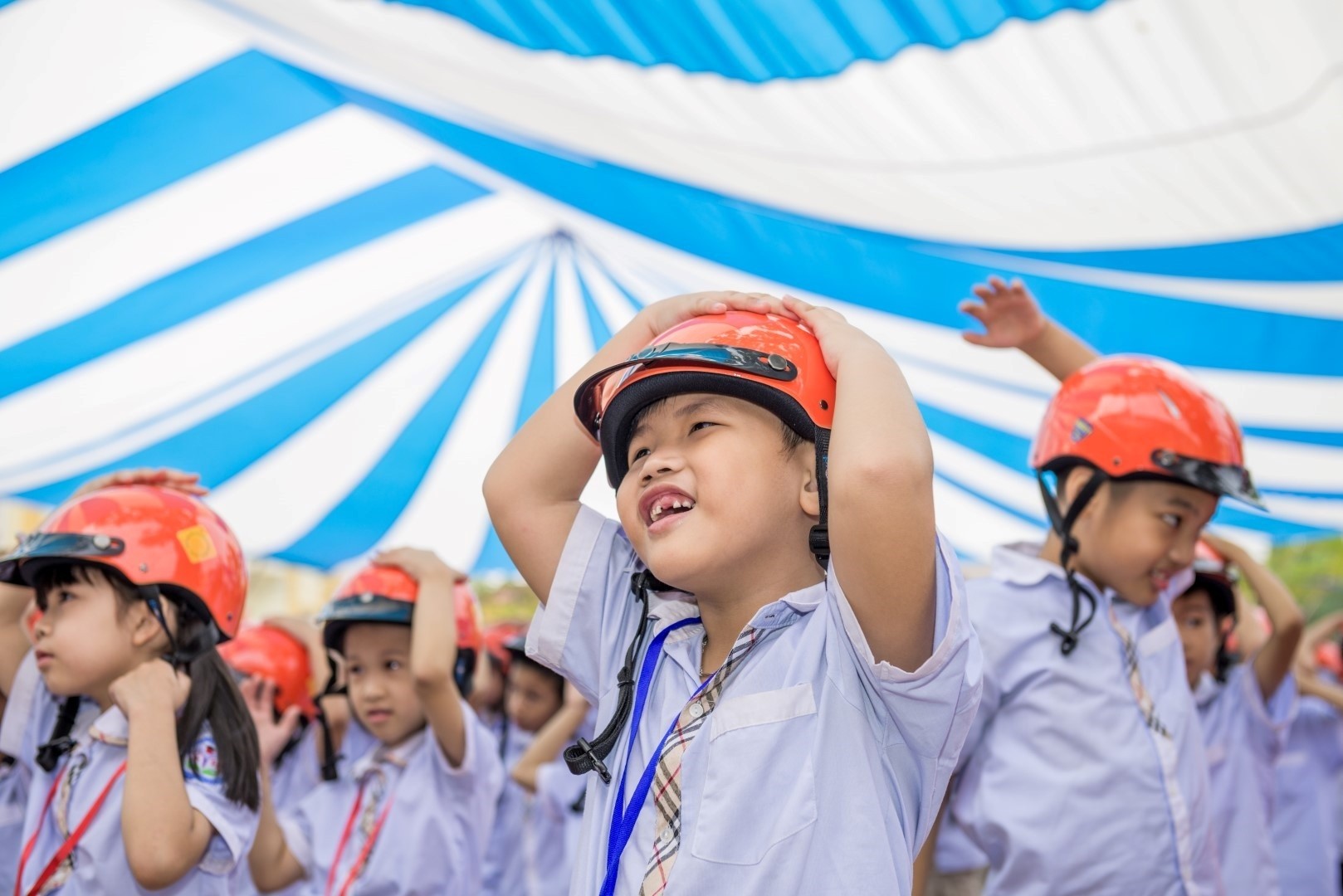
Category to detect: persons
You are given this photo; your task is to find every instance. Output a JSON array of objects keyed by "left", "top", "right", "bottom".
[
  {"left": 912, "top": 277, "right": 1265, "bottom": 892},
  {"left": 1175, "top": 529, "right": 1341, "bottom": 896},
  {"left": 1, "top": 471, "right": 581, "bottom": 896},
  {"left": 480, "top": 291, "right": 984, "bottom": 896}
]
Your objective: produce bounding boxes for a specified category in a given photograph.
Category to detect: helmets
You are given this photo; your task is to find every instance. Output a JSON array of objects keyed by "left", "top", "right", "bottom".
[
  {"left": 1032, "top": 354, "right": 1271, "bottom": 514},
  {"left": 1224, "top": 606, "right": 1275, "bottom": 657},
  {"left": 1192, "top": 538, "right": 1237, "bottom": 587},
  {"left": 215, "top": 626, "right": 322, "bottom": 718},
  {"left": 502, "top": 631, "right": 551, "bottom": 652},
  {"left": 313, "top": 559, "right": 485, "bottom": 690},
  {"left": 570, "top": 310, "right": 837, "bottom": 497},
  {"left": 0, "top": 484, "right": 249, "bottom": 645},
  {"left": 484, "top": 623, "right": 529, "bottom": 673},
  {"left": 1315, "top": 639, "right": 1343, "bottom": 683},
  {"left": 451, "top": 582, "right": 489, "bottom": 659}
]
